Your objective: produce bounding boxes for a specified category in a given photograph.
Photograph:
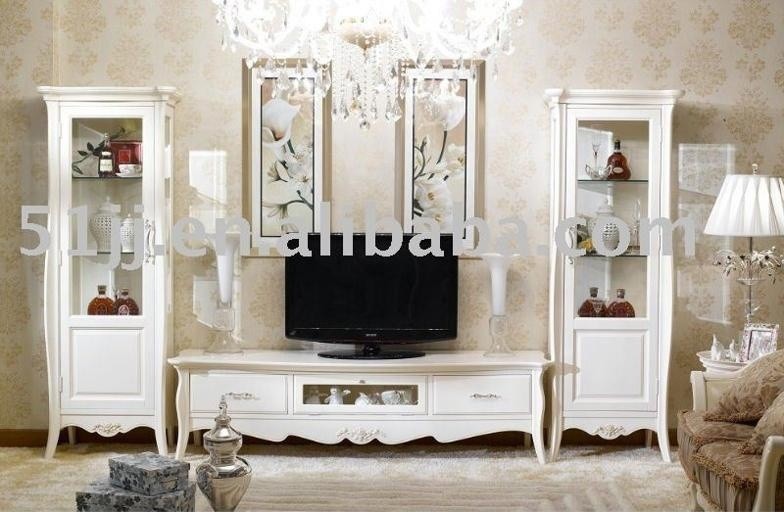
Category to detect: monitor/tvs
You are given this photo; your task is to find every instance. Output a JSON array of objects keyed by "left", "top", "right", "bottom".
[{"left": 283, "top": 230, "right": 459, "bottom": 340}]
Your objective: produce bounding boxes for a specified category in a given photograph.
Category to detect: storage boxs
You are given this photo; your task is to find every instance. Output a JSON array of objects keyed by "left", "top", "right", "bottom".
[{"left": 75, "top": 473, "right": 197, "bottom": 512}]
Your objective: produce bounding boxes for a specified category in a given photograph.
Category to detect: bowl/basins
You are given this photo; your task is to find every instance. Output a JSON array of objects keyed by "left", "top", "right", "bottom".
[{"left": 118, "top": 163, "right": 141, "bottom": 174}]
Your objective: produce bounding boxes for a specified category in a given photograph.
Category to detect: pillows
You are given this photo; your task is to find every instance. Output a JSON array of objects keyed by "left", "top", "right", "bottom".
[
  {"left": 703, "top": 348, "right": 784, "bottom": 419},
  {"left": 736, "top": 389, "right": 784, "bottom": 453}
]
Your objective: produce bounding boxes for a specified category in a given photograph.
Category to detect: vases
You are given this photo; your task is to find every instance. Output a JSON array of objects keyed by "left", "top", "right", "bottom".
[
  {"left": 478, "top": 253, "right": 522, "bottom": 358},
  {"left": 202, "top": 230, "right": 247, "bottom": 354}
]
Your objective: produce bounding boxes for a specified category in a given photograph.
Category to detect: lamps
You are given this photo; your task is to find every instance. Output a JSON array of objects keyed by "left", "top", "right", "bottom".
[
  {"left": 212, "top": 0, "right": 525, "bottom": 132},
  {"left": 702, "top": 174, "right": 783, "bottom": 322}
]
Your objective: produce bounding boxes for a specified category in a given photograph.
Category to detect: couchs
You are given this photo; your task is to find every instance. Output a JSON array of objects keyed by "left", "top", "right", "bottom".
[{"left": 676, "top": 350, "right": 784, "bottom": 511}]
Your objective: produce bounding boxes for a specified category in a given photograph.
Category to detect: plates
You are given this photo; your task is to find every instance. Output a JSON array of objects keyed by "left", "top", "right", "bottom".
[{"left": 115, "top": 173, "right": 142, "bottom": 178}]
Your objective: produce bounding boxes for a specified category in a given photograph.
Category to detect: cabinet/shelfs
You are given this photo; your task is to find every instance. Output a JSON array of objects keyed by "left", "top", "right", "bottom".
[
  {"left": 546, "top": 88, "right": 685, "bottom": 466},
  {"left": 35, "top": 84, "right": 182, "bottom": 461},
  {"left": 167, "top": 347, "right": 553, "bottom": 466}
]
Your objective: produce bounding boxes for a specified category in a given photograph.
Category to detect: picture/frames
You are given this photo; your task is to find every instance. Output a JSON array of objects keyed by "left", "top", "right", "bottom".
[
  {"left": 394, "top": 58, "right": 487, "bottom": 259},
  {"left": 739, "top": 323, "right": 779, "bottom": 362},
  {"left": 240, "top": 57, "right": 334, "bottom": 258}
]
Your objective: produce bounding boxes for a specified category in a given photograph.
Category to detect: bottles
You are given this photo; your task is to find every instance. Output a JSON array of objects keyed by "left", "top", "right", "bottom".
[
  {"left": 98, "top": 128, "right": 115, "bottom": 177},
  {"left": 577, "top": 287, "right": 607, "bottom": 318},
  {"left": 607, "top": 139, "right": 630, "bottom": 180},
  {"left": 88, "top": 284, "right": 114, "bottom": 314},
  {"left": 113, "top": 288, "right": 139, "bottom": 316},
  {"left": 607, "top": 289, "right": 635, "bottom": 317}
]
